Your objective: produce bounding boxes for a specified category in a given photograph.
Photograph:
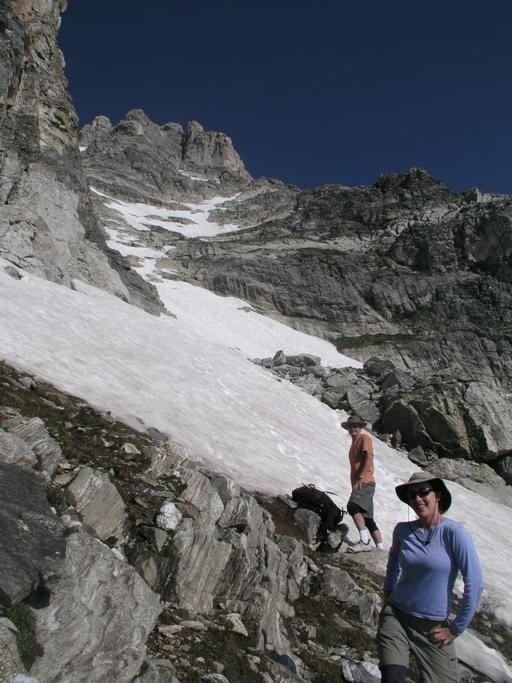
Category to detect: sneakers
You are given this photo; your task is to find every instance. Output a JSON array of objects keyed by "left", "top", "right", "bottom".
[{"left": 348, "top": 538, "right": 372, "bottom": 552}]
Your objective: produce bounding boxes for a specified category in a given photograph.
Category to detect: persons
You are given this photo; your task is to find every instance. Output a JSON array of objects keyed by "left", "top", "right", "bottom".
[
  {"left": 375, "top": 472, "right": 484, "bottom": 683},
  {"left": 340, "top": 415, "right": 384, "bottom": 554}
]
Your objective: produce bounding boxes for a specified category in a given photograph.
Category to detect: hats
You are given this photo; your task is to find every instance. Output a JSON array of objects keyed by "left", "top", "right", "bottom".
[
  {"left": 341, "top": 415, "right": 367, "bottom": 430},
  {"left": 395, "top": 471, "right": 451, "bottom": 514}
]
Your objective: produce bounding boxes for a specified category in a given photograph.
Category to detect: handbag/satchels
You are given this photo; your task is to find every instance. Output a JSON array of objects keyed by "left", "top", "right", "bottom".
[{"left": 292, "top": 485, "right": 342, "bottom": 530}]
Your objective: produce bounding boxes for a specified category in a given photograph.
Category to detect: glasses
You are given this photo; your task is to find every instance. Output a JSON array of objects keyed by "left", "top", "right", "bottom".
[{"left": 408, "top": 487, "right": 436, "bottom": 499}]
